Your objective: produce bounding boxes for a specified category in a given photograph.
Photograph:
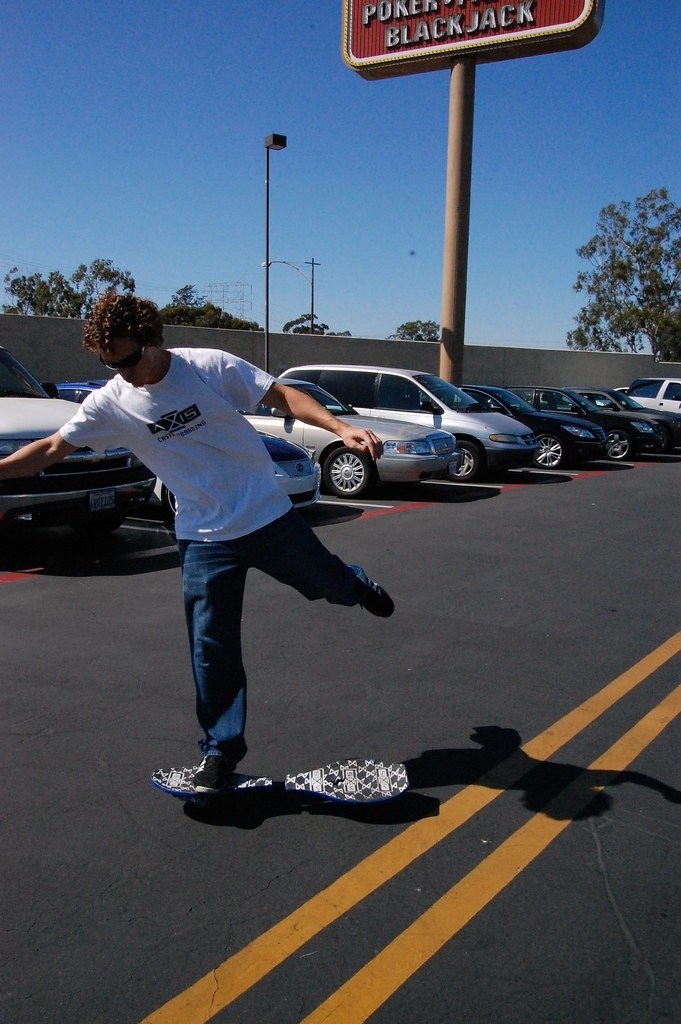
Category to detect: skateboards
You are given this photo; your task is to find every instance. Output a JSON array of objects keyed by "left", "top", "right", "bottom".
[{"left": 148, "top": 757, "right": 410, "bottom": 803}]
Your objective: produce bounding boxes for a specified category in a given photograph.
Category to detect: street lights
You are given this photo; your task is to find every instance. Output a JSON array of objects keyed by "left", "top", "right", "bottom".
[
  {"left": 261, "top": 256, "right": 322, "bottom": 333},
  {"left": 261, "top": 133, "right": 289, "bottom": 374}
]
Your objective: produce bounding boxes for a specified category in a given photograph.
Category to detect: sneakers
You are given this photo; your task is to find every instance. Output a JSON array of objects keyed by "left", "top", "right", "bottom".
[
  {"left": 193, "top": 746, "right": 237, "bottom": 793},
  {"left": 345, "top": 569, "right": 398, "bottom": 619}
]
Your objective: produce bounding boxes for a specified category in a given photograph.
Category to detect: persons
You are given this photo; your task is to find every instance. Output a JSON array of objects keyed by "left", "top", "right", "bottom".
[{"left": 0, "top": 295, "right": 394, "bottom": 787}]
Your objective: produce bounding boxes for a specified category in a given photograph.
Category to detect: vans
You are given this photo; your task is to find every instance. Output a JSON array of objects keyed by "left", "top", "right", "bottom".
[
  {"left": 622, "top": 377, "right": 681, "bottom": 413},
  {"left": 278, "top": 363, "right": 540, "bottom": 484}
]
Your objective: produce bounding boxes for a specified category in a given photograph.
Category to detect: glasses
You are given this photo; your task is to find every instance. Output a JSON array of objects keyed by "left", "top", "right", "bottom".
[{"left": 99, "top": 343, "right": 145, "bottom": 369}]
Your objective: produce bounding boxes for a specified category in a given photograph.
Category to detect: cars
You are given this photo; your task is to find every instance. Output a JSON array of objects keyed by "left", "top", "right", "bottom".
[
  {"left": 55, "top": 379, "right": 322, "bottom": 523},
  {"left": 583, "top": 387, "right": 631, "bottom": 407},
  {"left": 237, "top": 379, "right": 467, "bottom": 499},
  {"left": 426, "top": 384, "right": 612, "bottom": 471},
  {"left": 490, "top": 385, "right": 665, "bottom": 461},
  {"left": 540, "top": 386, "right": 681, "bottom": 454}
]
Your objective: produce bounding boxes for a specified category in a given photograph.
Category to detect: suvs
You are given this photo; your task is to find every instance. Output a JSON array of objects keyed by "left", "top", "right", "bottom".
[{"left": 0, "top": 345, "right": 157, "bottom": 539}]
